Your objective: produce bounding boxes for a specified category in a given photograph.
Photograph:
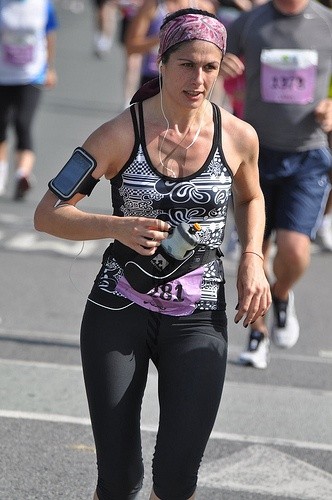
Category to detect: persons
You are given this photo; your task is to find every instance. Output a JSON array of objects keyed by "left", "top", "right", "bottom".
[
  {"left": 0, "top": 0, "right": 59, "bottom": 201},
  {"left": 222, "top": 0, "right": 332, "bottom": 370},
  {"left": 34, "top": 7, "right": 273, "bottom": 500},
  {"left": 92, "top": 0, "right": 332, "bottom": 258}
]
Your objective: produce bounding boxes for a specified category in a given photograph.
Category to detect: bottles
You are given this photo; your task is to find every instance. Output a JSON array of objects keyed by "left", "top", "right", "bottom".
[{"left": 123, "top": 222, "right": 201, "bottom": 294}]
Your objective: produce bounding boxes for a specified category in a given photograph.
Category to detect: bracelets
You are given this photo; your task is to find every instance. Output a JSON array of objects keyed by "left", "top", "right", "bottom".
[{"left": 242, "top": 251, "right": 264, "bottom": 262}]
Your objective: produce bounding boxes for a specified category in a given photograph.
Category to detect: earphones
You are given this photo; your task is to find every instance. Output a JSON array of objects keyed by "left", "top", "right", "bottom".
[{"left": 159, "top": 60, "right": 163, "bottom": 69}]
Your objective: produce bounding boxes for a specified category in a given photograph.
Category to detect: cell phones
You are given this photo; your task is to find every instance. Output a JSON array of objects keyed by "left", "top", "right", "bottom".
[{"left": 48, "top": 147, "right": 97, "bottom": 200}]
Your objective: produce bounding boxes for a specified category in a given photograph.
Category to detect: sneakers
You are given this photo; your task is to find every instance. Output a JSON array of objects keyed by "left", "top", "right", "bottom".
[
  {"left": 269, "top": 288, "right": 299, "bottom": 350},
  {"left": 239, "top": 329, "right": 270, "bottom": 368}
]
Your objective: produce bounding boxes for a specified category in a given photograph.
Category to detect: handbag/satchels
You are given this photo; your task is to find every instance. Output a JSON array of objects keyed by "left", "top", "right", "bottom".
[{"left": 109, "top": 238, "right": 225, "bottom": 294}]
